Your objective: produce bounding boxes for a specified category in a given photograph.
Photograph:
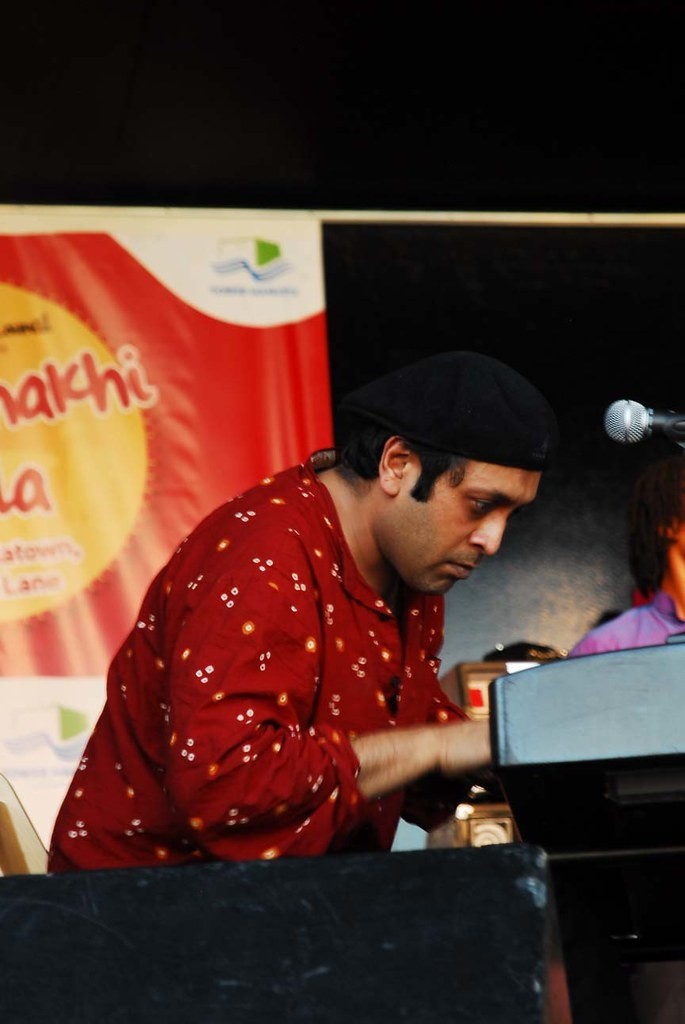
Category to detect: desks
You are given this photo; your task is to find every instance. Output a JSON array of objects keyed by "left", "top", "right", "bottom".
[{"left": 0, "top": 839, "right": 684, "bottom": 1024}]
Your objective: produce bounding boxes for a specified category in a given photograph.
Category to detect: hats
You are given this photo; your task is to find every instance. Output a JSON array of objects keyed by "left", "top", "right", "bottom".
[{"left": 337, "top": 350, "right": 559, "bottom": 471}]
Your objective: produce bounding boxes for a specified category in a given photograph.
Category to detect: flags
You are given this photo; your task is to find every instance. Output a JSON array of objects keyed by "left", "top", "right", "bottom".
[{"left": 0, "top": 219, "right": 333, "bottom": 874}]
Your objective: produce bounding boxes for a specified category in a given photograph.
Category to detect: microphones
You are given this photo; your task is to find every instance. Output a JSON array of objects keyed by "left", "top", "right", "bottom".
[{"left": 605, "top": 400, "right": 685, "bottom": 445}]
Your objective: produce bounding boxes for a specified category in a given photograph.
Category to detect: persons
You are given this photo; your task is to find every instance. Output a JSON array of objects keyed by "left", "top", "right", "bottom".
[
  {"left": 49, "top": 351, "right": 574, "bottom": 1024},
  {"left": 568, "top": 485, "right": 685, "bottom": 1024}
]
